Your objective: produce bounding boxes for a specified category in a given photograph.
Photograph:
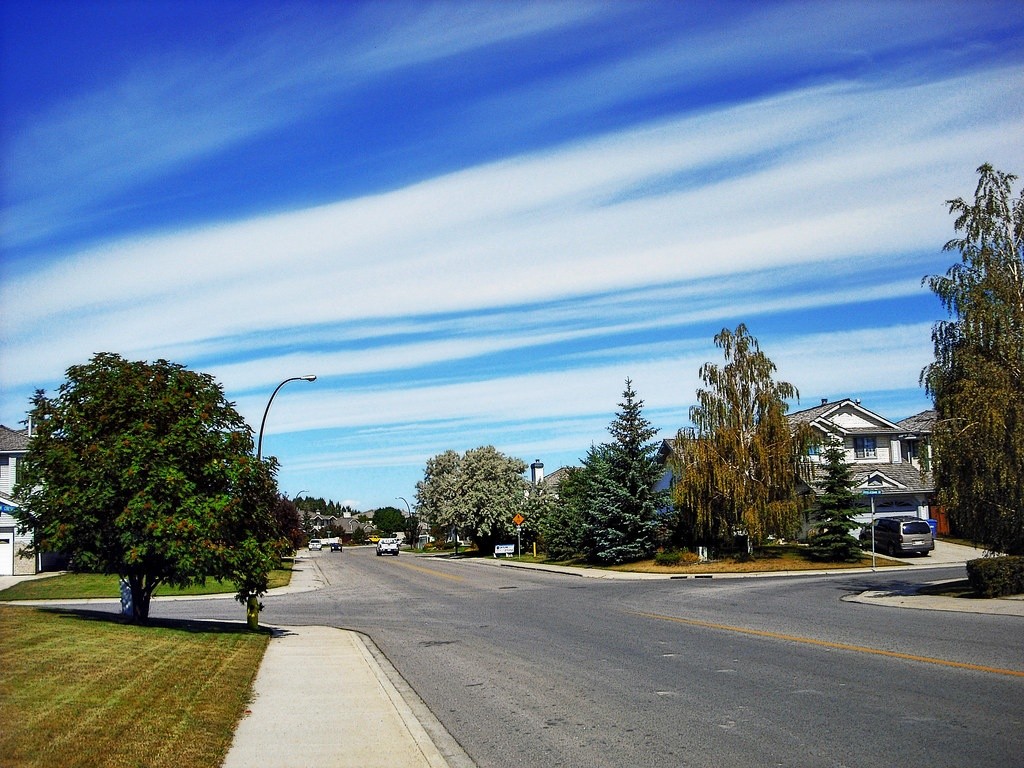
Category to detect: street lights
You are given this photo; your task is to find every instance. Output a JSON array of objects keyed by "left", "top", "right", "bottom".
[
  {"left": 258, "top": 374, "right": 315, "bottom": 463},
  {"left": 395, "top": 496, "right": 411, "bottom": 518}
]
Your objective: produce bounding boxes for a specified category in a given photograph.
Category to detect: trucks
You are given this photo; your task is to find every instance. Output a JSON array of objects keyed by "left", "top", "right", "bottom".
[{"left": 391, "top": 532, "right": 410, "bottom": 542}]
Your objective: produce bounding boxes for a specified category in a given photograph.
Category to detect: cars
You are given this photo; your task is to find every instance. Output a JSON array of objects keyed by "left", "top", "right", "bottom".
[
  {"left": 330, "top": 543, "right": 343, "bottom": 553},
  {"left": 308, "top": 538, "right": 323, "bottom": 550},
  {"left": 395, "top": 538, "right": 402, "bottom": 547}
]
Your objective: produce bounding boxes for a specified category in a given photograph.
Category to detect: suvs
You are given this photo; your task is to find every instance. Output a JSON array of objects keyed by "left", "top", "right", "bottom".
[
  {"left": 859, "top": 516, "right": 934, "bottom": 556},
  {"left": 367, "top": 535, "right": 380, "bottom": 543},
  {"left": 376, "top": 538, "right": 399, "bottom": 556}
]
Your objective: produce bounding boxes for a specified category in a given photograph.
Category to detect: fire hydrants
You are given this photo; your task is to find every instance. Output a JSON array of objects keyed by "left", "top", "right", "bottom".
[{"left": 247, "top": 595, "right": 265, "bottom": 630}]
